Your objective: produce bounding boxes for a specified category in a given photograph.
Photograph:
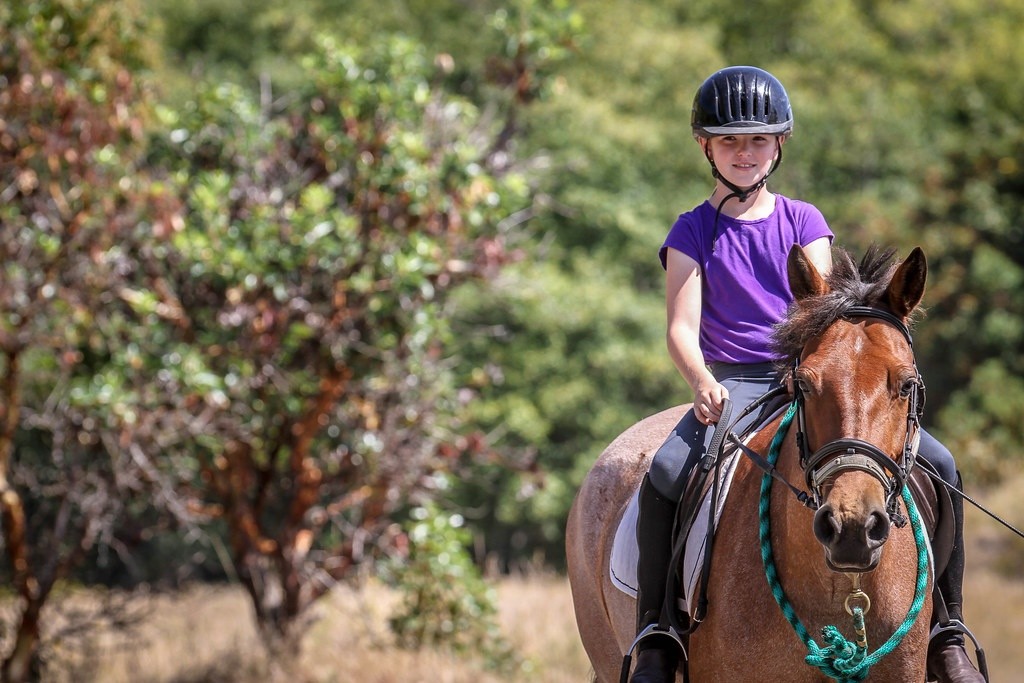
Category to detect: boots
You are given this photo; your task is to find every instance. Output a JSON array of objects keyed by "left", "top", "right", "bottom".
[
  {"left": 625, "top": 472, "right": 683, "bottom": 683},
  {"left": 929, "top": 471, "right": 988, "bottom": 683}
]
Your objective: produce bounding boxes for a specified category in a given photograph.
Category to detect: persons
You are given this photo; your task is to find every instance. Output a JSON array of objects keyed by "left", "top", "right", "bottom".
[{"left": 616, "top": 66, "right": 986, "bottom": 683}]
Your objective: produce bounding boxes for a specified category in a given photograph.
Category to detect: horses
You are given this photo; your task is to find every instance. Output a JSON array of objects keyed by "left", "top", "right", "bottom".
[{"left": 562, "top": 240, "right": 934, "bottom": 683}]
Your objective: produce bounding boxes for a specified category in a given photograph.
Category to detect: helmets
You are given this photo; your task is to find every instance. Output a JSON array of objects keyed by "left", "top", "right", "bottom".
[{"left": 691, "top": 66, "right": 794, "bottom": 140}]
